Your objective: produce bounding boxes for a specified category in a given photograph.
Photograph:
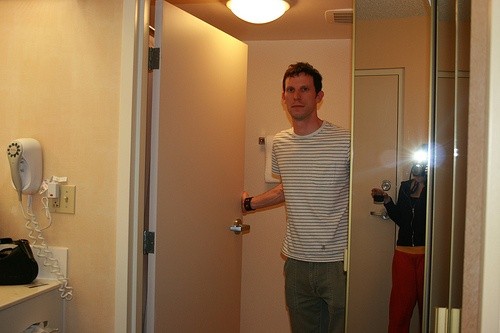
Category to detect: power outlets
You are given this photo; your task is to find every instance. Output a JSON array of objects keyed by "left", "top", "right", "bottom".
[{"left": 47, "top": 185, "right": 74, "bottom": 214}]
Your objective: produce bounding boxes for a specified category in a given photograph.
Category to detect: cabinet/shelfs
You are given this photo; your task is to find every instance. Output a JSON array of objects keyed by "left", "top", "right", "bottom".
[{"left": 0, "top": 244, "right": 70, "bottom": 333}]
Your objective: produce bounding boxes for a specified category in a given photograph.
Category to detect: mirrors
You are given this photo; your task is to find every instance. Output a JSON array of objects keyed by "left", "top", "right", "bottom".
[{"left": 354, "top": 0, "right": 430, "bottom": 333}]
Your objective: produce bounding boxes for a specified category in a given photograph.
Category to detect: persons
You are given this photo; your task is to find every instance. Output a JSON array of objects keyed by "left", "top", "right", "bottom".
[
  {"left": 371, "top": 144, "right": 428, "bottom": 333},
  {"left": 241, "top": 63, "right": 351, "bottom": 333}
]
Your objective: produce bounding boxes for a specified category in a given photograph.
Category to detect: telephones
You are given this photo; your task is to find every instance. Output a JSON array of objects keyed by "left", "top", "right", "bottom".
[{"left": 7, "top": 137, "right": 43, "bottom": 202}]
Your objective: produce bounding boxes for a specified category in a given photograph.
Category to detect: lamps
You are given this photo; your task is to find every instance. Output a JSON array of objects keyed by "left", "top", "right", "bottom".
[{"left": 225, "top": 0, "right": 290, "bottom": 24}]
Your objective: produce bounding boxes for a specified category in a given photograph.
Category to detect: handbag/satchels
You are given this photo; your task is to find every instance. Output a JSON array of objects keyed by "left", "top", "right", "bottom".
[{"left": 0, "top": 238, "right": 39, "bottom": 286}]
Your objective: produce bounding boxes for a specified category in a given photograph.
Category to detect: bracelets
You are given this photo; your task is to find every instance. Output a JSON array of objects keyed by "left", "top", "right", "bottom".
[{"left": 244, "top": 197, "right": 257, "bottom": 211}]
